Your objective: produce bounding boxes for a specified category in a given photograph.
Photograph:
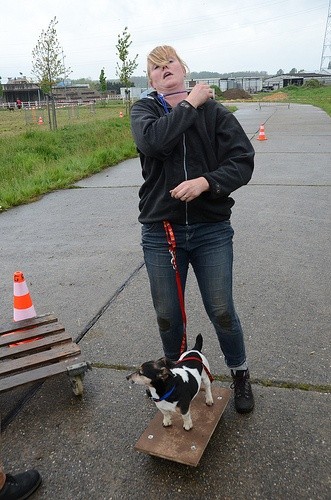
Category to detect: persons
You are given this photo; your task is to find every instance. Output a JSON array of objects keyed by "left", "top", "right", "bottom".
[
  {"left": 16, "top": 99, "right": 22, "bottom": 109},
  {"left": 131, "top": 46, "right": 255, "bottom": 413}
]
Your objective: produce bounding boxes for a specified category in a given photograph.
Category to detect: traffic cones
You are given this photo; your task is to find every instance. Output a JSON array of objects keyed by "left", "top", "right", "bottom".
[
  {"left": 119, "top": 111, "right": 123, "bottom": 117},
  {"left": 257, "top": 123, "right": 268, "bottom": 141},
  {"left": 37, "top": 116, "right": 43, "bottom": 125},
  {"left": 6, "top": 271, "right": 44, "bottom": 352}
]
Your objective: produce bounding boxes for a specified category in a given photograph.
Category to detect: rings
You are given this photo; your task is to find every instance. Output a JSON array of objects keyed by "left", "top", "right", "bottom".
[{"left": 185, "top": 195, "right": 188, "bottom": 199}]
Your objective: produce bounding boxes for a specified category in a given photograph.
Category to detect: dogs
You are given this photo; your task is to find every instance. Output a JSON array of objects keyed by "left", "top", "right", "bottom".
[{"left": 126, "top": 333, "right": 214, "bottom": 430}]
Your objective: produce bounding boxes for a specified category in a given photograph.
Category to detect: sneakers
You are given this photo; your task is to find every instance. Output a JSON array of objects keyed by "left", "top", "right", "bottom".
[
  {"left": 230, "top": 368, "right": 254, "bottom": 416},
  {"left": 145, "top": 389, "right": 151, "bottom": 396},
  {"left": 0, "top": 467, "right": 43, "bottom": 499}
]
